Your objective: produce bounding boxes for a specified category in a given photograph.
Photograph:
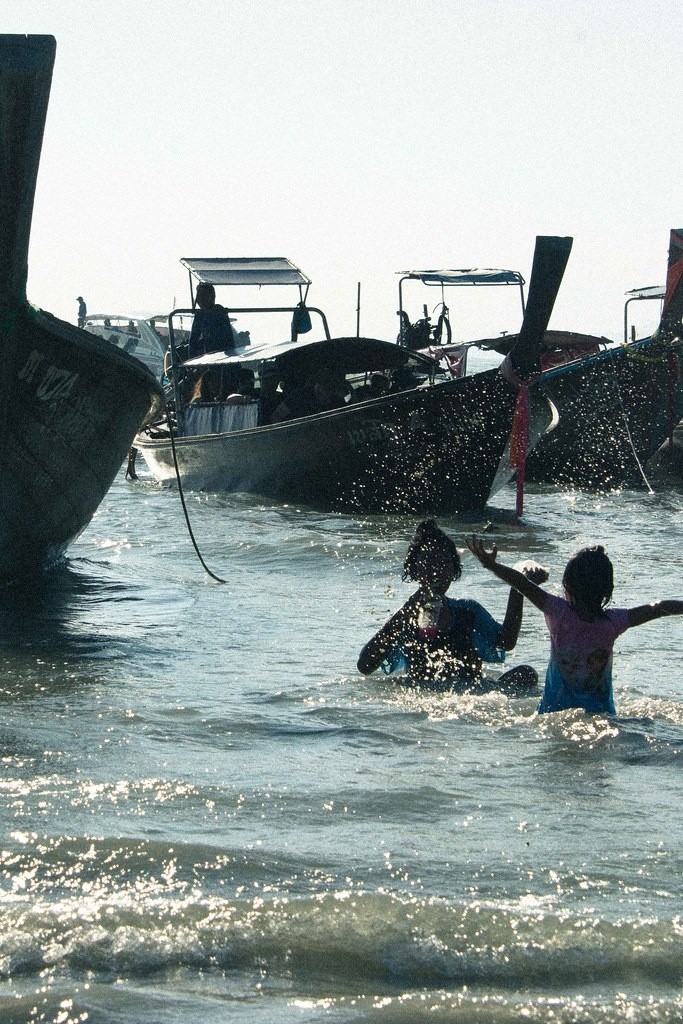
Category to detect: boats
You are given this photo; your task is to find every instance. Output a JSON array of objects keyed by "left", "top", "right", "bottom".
[
  {"left": 1, "top": 32, "right": 166, "bottom": 596},
  {"left": 129, "top": 235, "right": 575, "bottom": 524},
  {"left": 379, "top": 229, "right": 683, "bottom": 493}
]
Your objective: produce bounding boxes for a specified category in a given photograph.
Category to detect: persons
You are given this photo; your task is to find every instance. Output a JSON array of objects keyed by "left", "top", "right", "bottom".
[
  {"left": 357, "top": 518, "right": 549, "bottom": 684},
  {"left": 188, "top": 284, "right": 254, "bottom": 407},
  {"left": 464, "top": 533, "right": 683, "bottom": 718},
  {"left": 76, "top": 297, "right": 87, "bottom": 329}
]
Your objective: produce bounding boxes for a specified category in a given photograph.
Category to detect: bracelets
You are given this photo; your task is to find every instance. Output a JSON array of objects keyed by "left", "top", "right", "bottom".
[{"left": 482, "top": 560, "right": 495, "bottom": 568}]
